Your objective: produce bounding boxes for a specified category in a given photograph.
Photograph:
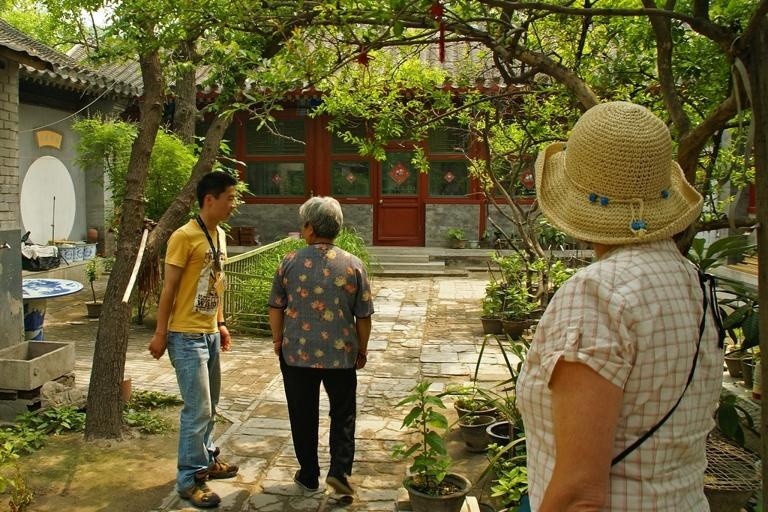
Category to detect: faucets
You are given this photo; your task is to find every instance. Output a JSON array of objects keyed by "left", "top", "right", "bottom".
[{"left": 0, "top": 240, "right": 12, "bottom": 250}]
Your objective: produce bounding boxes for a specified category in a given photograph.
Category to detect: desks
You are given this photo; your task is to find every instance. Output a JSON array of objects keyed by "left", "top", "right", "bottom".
[{"left": 20, "top": 277, "right": 83, "bottom": 343}]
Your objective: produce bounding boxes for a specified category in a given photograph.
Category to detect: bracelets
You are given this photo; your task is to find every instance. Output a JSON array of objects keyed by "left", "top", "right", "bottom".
[{"left": 217, "top": 322, "right": 226, "bottom": 327}]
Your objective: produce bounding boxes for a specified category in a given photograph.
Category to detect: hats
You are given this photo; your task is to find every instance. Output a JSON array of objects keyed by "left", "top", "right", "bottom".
[{"left": 531, "top": 99, "right": 706, "bottom": 248}]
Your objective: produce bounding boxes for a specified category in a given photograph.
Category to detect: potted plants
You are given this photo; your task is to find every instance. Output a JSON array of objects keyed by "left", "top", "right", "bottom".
[
  {"left": 443, "top": 225, "right": 578, "bottom": 250},
  {"left": 81, "top": 254, "right": 107, "bottom": 320},
  {"left": 387, "top": 378, "right": 532, "bottom": 512},
  {"left": 711, "top": 287, "right": 762, "bottom": 404},
  {"left": 480, "top": 256, "right": 571, "bottom": 340}
]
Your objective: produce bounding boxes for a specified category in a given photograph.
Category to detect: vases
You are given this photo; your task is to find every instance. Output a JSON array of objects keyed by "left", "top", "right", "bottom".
[
  {"left": 73, "top": 244, "right": 85, "bottom": 261},
  {"left": 57, "top": 245, "right": 74, "bottom": 264},
  {"left": 84, "top": 243, "right": 97, "bottom": 260}
]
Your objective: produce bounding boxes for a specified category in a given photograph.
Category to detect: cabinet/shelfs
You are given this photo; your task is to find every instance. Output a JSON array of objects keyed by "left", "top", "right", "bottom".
[{"left": 701, "top": 262, "right": 763, "bottom": 411}]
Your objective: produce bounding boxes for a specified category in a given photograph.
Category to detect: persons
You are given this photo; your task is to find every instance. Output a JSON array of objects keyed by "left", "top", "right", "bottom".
[
  {"left": 515, "top": 102, "right": 725, "bottom": 511},
  {"left": 149, "top": 172, "right": 239, "bottom": 508},
  {"left": 268, "top": 196, "right": 374, "bottom": 495}
]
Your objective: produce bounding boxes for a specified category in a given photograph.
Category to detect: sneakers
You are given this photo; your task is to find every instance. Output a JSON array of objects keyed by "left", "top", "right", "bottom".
[
  {"left": 177, "top": 469, "right": 221, "bottom": 508},
  {"left": 207, "top": 447, "right": 239, "bottom": 479},
  {"left": 294, "top": 470, "right": 320, "bottom": 492},
  {"left": 325, "top": 469, "right": 355, "bottom": 496}
]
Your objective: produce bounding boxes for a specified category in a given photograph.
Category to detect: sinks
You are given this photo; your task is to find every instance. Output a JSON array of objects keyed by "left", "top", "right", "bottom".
[{"left": 0, "top": 337, "right": 76, "bottom": 391}]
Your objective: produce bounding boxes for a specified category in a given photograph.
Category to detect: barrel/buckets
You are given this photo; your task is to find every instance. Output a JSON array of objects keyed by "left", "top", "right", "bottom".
[
  {"left": 240, "top": 224, "right": 256, "bottom": 245},
  {"left": 226, "top": 226, "right": 240, "bottom": 246}
]
